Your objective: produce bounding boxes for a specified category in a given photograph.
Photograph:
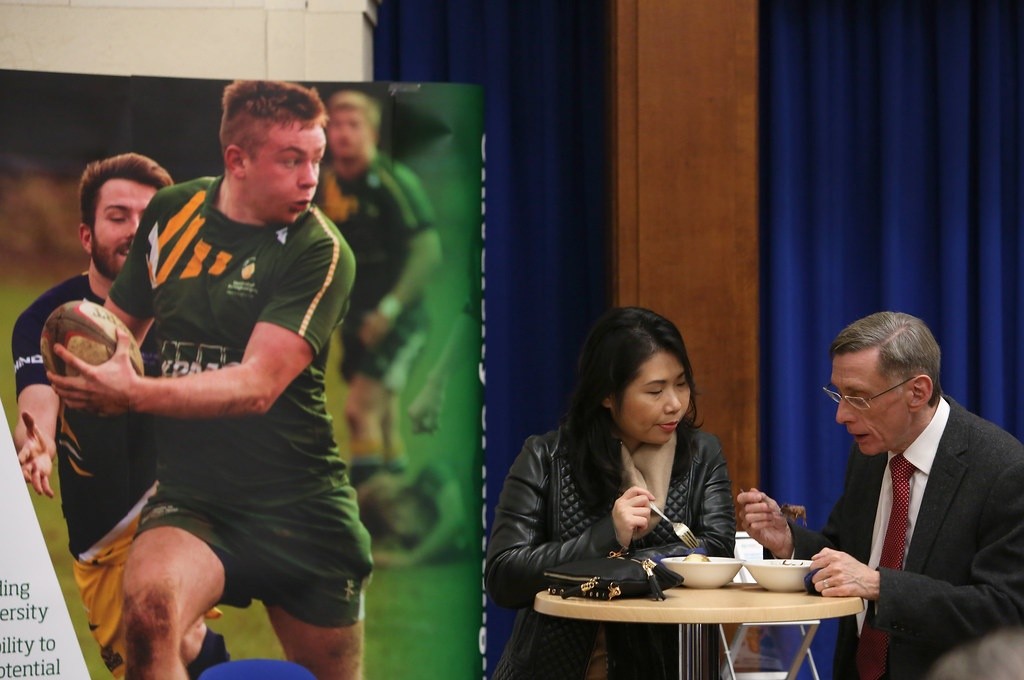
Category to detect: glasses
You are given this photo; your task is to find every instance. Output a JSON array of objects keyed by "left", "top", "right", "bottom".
[{"left": 822, "top": 376, "right": 917, "bottom": 410}]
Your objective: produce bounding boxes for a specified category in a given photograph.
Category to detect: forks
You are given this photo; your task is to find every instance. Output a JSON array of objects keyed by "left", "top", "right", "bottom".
[{"left": 650, "top": 501, "right": 700, "bottom": 552}]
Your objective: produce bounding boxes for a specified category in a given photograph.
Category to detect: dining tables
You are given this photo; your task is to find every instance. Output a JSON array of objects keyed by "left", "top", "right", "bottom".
[{"left": 534, "top": 581, "right": 865, "bottom": 680}]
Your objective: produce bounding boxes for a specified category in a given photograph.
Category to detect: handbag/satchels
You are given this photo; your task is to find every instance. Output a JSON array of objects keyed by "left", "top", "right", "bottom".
[{"left": 542, "top": 551, "right": 684, "bottom": 601}]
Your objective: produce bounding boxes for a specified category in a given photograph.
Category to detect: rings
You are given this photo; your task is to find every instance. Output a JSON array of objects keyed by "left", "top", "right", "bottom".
[{"left": 823, "top": 579, "right": 829, "bottom": 588}]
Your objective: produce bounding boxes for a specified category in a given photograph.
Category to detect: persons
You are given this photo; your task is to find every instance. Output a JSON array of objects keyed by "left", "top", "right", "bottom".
[
  {"left": 736, "top": 310, "right": 1024, "bottom": 680},
  {"left": 12, "top": 81, "right": 485, "bottom": 680},
  {"left": 485, "top": 306, "right": 737, "bottom": 680}
]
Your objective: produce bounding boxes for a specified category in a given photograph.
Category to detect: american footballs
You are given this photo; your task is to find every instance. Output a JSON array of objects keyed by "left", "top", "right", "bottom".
[{"left": 40, "top": 298, "right": 145, "bottom": 418}]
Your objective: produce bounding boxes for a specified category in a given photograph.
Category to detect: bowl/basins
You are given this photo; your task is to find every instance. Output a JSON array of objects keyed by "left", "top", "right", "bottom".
[
  {"left": 743, "top": 559, "right": 816, "bottom": 592},
  {"left": 661, "top": 558, "right": 745, "bottom": 589}
]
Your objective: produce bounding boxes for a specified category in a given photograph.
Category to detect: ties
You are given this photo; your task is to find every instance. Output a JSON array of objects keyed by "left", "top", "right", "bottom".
[{"left": 855, "top": 454, "right": 918, "bottom": 680}]
